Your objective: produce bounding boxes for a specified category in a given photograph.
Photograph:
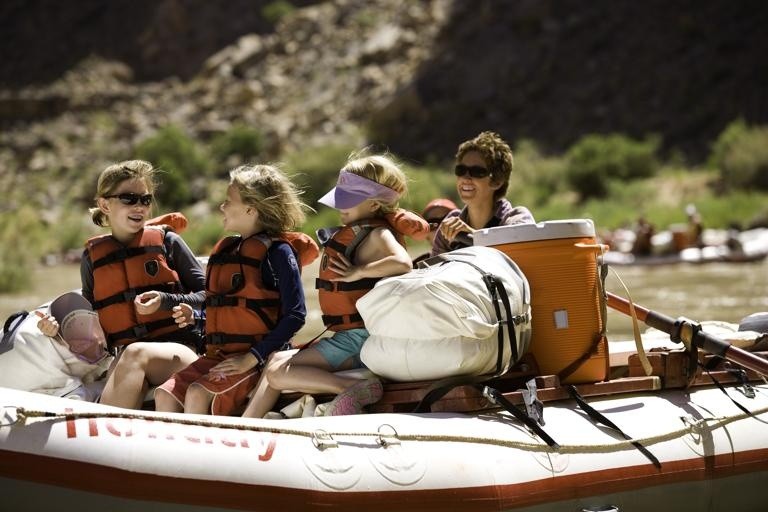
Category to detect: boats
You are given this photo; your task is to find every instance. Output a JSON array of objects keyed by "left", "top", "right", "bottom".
[
  {"left": 0, "top": 311, "right": 767, "bottom": 512},
  {"left": 566, "top": 223, "right": 768, "bottom": 265}
]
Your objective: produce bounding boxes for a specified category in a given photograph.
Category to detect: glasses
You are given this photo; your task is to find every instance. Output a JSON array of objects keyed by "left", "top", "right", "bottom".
[
  {"left": 104, "top": 191, "right": 153, "bottom": 207},
  {"left": 455, "top": 164, "right": 493, "bottom": 179},
  {"left": 315, "top": 225, "right": 342, "bottom": 246}
]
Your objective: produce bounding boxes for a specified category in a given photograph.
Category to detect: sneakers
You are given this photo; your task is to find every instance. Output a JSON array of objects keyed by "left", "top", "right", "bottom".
[{"left": 324, "top": 377, "right": 384, "bottom": 416}]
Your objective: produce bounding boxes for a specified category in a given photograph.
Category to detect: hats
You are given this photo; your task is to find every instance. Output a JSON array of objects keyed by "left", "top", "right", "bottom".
[
  {"left": 317, "top": 167, "right": 401, "bottom": 210},
  {"left": 49, "top": 292, "right": 109, "bottom": 364},
  {"left": 422, "top": 199, "right": 458, "bottom": 218}
]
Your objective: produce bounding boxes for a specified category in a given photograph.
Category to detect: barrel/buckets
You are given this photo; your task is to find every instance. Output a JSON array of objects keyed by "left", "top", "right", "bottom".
[{"left": 472, "top": 218, "right": 608, "bottom": 384}]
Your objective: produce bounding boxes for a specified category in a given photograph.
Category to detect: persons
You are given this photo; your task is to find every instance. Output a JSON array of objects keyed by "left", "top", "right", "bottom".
[
  {"left": 428, "top": 130, "right": 535, "bottom": 255},
  {"left": 35, "top": 160, "right": 207, "bottom": 410},
  {"left": 597, "top": 204, "right": 703, "bottom": 259},
  {"left": 413, "top": 197, "right": 457, "bottom": 270},
  {"left": 242, "top": 143, "right": 430, "bottom": 418},
  {"left": 151, "top": 163, "right": 317, "bottom": 417}
]
